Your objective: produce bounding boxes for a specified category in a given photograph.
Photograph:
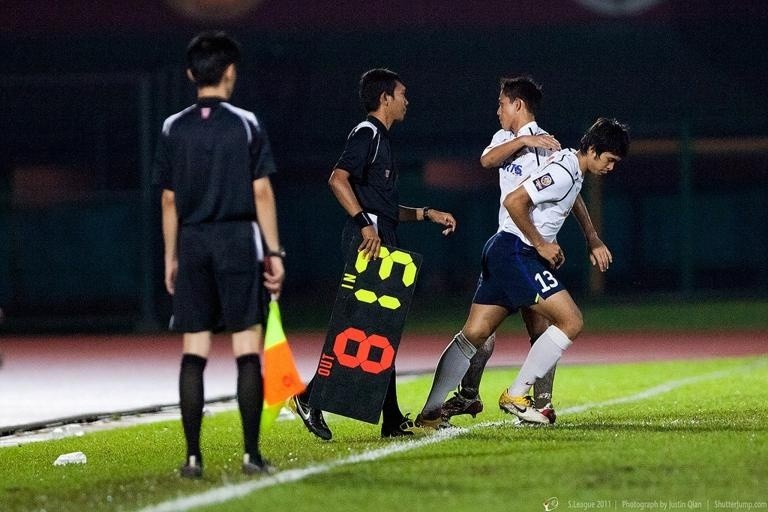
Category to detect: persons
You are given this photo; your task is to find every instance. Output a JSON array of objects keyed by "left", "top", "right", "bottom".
[
  {"left": 413, "top": 116, "right": 631, "bottom": 432},
  {"left": 441, "top": 74, "right": 613, "bottom": 426},
  {"left": 154, "top": 27, "right": 292, "bottom": 478},
  {"left": 286, "top": 67, "right": 458, "bottom": 441}
]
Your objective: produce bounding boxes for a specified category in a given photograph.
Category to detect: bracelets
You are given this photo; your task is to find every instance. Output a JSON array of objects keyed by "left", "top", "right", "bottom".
[
  {"left": 422, "top": 205, "right": 433, "bottom": 221},
  {"left": 352, "top": 209, "right": 374, "bottom": 229},
  {"left": 266, "top": 244, "right": 287, "bottom": 258}
]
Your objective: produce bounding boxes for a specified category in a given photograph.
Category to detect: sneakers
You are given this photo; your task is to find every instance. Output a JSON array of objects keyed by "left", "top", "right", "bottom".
[
  {"left": 182, "top": 456, "right": 201, "bottom": 479},
  {"left": 242, "top": 453, "right": 274, "bottom": 474},
  {"left": 287, "top": 384, "right": 556, "bottom": 440}
]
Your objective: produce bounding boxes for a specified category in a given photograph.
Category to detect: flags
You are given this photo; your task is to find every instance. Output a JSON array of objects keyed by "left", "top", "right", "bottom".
[{"left": 256, "top": 299, "right": 305, "bottom": 437}]
